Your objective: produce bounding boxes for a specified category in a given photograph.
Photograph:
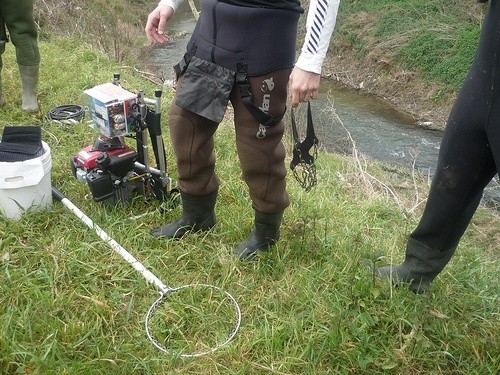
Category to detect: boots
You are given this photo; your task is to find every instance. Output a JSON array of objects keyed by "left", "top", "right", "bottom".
[
  {"left": 372, "top": 237, "right": 458, "bottom": 292},
  {"left": 233, "top": 205, "right": 284, "bottom": 261},
  {"left": 150, "top": 186, "right": 220, "bottom": 241},
  {"left": 0, "top": 68, "right": 39, "bottom": 112}
]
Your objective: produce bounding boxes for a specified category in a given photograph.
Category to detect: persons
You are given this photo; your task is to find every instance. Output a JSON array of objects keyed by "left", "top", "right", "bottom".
[
  {"left": 144, "top": 0, "right": 340, "bottom": 262},
  {"left": 0, "top": 0, "right": 39, "bottom": 113},
  {"left": 376, "top": 0, "right": 500, "bottom": 294}
]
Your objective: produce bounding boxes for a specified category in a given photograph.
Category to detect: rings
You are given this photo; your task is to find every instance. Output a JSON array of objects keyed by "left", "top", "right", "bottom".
[{"left": 155, "top": 28, "right": 158, "bottom": 30}]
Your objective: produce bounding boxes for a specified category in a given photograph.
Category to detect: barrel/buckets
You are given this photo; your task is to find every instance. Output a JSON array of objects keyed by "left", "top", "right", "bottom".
[{"left": 0, "top": 141, "right": 54, "bottom": 219}]
